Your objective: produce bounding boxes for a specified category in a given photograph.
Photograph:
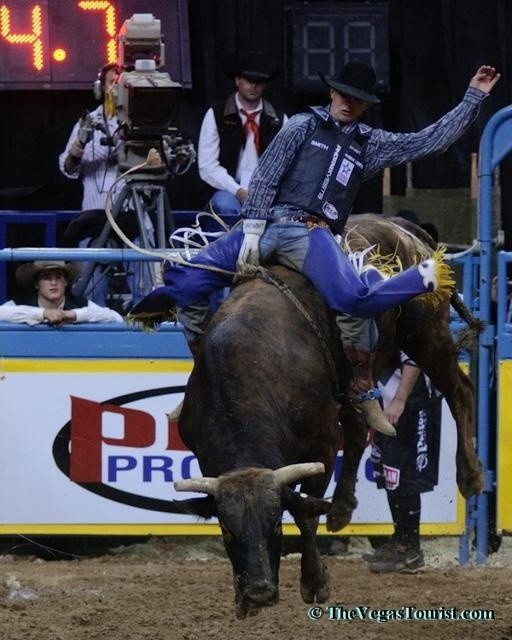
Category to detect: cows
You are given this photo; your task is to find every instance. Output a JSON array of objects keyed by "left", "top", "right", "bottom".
[{"left": 173, "top": 213, "right": 490, "bottom": 621}]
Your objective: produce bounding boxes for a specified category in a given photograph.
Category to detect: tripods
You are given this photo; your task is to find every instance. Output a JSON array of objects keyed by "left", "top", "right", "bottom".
[{"left": 72, "top": 176, "right": 177, "bottom": 299}]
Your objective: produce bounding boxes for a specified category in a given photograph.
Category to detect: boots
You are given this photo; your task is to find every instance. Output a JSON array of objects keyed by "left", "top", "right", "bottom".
[{"left": 342, "top": 342, "right": 398, "bottom": 437}]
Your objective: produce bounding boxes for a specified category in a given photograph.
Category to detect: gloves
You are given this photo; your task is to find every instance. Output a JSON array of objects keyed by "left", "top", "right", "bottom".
[{"left": 77, "top": 114, "right": 94, "bottom": 144}]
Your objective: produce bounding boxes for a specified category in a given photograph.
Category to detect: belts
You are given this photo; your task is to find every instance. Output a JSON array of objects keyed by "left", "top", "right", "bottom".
[{"left": 268, "top": 216, "right": 324, "bottom": 227}]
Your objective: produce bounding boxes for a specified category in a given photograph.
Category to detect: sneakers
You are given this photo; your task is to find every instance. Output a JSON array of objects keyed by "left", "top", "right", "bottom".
[
  {"left": 362, "top": 543, "right": 392, "bottom": 563},
  {"left": 368, "top": 549, "right": 427, "bottom": 574}
]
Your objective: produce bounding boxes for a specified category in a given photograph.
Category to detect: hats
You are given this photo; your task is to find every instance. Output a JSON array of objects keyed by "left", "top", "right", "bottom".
[
  {"left": 316, "top": 60, "right": 382, "bottom": 104},
  {"left": 232, "top": 55, "right": 271, "bottom": 81},
  {"left": 14, "top": 258, "right": 81, "bottom": 293}
]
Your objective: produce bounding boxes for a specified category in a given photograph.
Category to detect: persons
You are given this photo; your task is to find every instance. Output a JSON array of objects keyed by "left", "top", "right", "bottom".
[
  {"left": 195, "top": 52, "right": 289, "bottom": 241},
  {"left": 0, "top": 260, "right": 127, "bottom": 562},
  {"left": 448, "top": 274, "right": 512, "bottom": 327},
  {"left": 59, "top": 62, "right": 157, "bottom": 320},
  {"left": 130, "top": 60, "right": 503, "bottom": 438},
  {"left": 361, "top": 334, "right": 448, "bottom": 576}
]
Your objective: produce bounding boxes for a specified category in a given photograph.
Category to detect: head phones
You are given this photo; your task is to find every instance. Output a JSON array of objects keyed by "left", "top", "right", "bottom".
[{"left": 94, "top": 62, "right": 118, "bottom": 100}]
